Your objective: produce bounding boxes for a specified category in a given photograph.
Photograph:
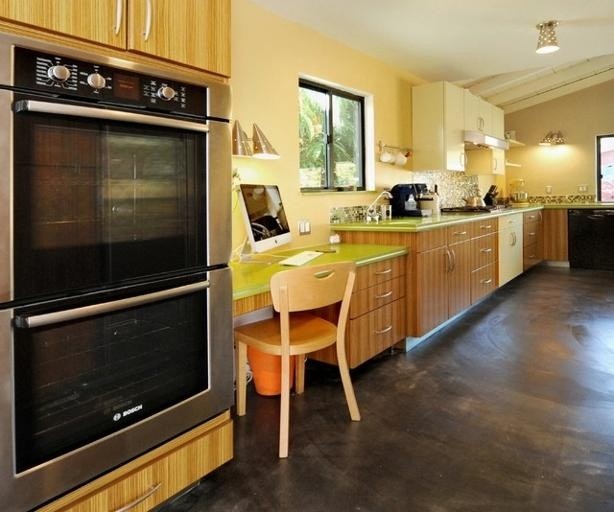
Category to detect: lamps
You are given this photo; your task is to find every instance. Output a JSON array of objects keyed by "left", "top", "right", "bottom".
[
  {"left": 231, "top": 119, "right": 280, "bottom": 161},
  {"left": 538, "top": 130, "right": 567, "bottom": 148},
  {"left": 534, "top": 17, "right": 563, "bottom": 56}
]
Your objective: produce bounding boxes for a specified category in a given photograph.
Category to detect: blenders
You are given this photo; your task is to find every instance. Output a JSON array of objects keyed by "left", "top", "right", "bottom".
[{"left": 508, "top": 178, "right": 530, "bottom": 208}]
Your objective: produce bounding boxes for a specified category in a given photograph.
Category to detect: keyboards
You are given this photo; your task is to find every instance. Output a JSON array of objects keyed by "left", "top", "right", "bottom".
[{"left": 278, "top": 251, "right": 324, "bottom": 267}]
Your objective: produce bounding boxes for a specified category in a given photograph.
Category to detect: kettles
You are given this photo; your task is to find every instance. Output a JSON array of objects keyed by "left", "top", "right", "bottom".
[{"left": 462, "top": 194, "right": 486, "bottom": 209}]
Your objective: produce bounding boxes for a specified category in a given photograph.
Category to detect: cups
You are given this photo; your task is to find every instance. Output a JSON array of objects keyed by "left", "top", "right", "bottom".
[
  {"left": 380, "top": 151, "right": 407, "bottom": 165},
  {"left": 379, "top": 204, "right": 392, "bottom": 220}
]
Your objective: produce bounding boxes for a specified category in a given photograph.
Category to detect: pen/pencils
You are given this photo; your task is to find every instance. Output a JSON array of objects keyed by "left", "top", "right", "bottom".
[{"left": 315, "top": 249, "right": 336, "bottom": 253}]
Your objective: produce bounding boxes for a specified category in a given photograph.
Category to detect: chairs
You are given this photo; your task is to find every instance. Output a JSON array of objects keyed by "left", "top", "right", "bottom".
[{"left": 232, "top": 261, "right": 360, "bottom": 460}]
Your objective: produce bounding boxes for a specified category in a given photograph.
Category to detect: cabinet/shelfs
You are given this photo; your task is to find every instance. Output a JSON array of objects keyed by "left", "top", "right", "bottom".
[
  {"left": 410, "top": 81, "right": 465, "bottom": 172},
  {"left": 465, "top": 89, "right": 494, "bottom": 138},
  {"left": 499, "top": 212, "right": 523, "bottom": 288},
  {"left": 1, "top": 1, "right": 234, "bottom": 82},
  {"left": 31, "top": 408, "right": 234, "bottom": 512},
  {"left": 542, "top": 208, "right": 569, "bottom": 267},
  {"left": 522, "top": 209, "right": 545, "bottom": 274},
  {"left": 329, "top": 221, "right": 471, "bottom": 353},
  {"left": 472, "top": 217, "right": 499, "bottom": 303},
  {"left": 468, "top": 104, "right": 505, "bottom": 176},
  {"left": 506, "top": 139, "right": 526, "bottom": 168}
]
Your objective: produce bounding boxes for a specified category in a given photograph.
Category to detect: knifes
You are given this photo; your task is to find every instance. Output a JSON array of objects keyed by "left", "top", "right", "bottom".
[{"left": 489, "top": 185, "right": 499, "bottom": 197}]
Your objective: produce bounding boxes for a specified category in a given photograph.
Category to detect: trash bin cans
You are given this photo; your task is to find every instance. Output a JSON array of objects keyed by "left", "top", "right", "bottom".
[{"left": 246, "top": 344, "right": 295, "bottom": 396}]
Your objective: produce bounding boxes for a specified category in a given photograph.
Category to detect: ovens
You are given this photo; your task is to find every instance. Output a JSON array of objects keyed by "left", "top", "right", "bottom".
[{"left": 1, "top": 31, "right": 238, "bottom": 511}]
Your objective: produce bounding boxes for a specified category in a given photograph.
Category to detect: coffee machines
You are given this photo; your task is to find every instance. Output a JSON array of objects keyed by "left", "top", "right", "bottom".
[{"left": 390, "top": 182, "right": 440, "bottom": 216}]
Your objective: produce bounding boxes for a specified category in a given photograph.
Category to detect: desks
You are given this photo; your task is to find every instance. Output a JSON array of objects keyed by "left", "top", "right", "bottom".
[{"left": 232, "top": 243, "right": 409, "bottom": 371}]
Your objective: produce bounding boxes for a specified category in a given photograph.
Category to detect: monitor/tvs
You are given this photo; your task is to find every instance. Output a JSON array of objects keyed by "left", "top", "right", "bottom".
[{"left": 233, "top": 183, "right": 294, "bottom": 261}]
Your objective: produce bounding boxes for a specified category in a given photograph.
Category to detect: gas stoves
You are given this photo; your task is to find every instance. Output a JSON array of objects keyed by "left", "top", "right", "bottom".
[{"left": 441, "top": 206, "right": 502, "bottom": 213}]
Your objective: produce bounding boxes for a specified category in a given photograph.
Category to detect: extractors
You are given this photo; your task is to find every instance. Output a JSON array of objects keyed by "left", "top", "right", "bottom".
[{"left": 464, "top": 130, "right": 509, "bottom": 152}]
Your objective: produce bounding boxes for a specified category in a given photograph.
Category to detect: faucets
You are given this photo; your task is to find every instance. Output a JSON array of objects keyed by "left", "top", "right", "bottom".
[{"left": 364, "top": 190, "right": 394, "bottom": 224}]
